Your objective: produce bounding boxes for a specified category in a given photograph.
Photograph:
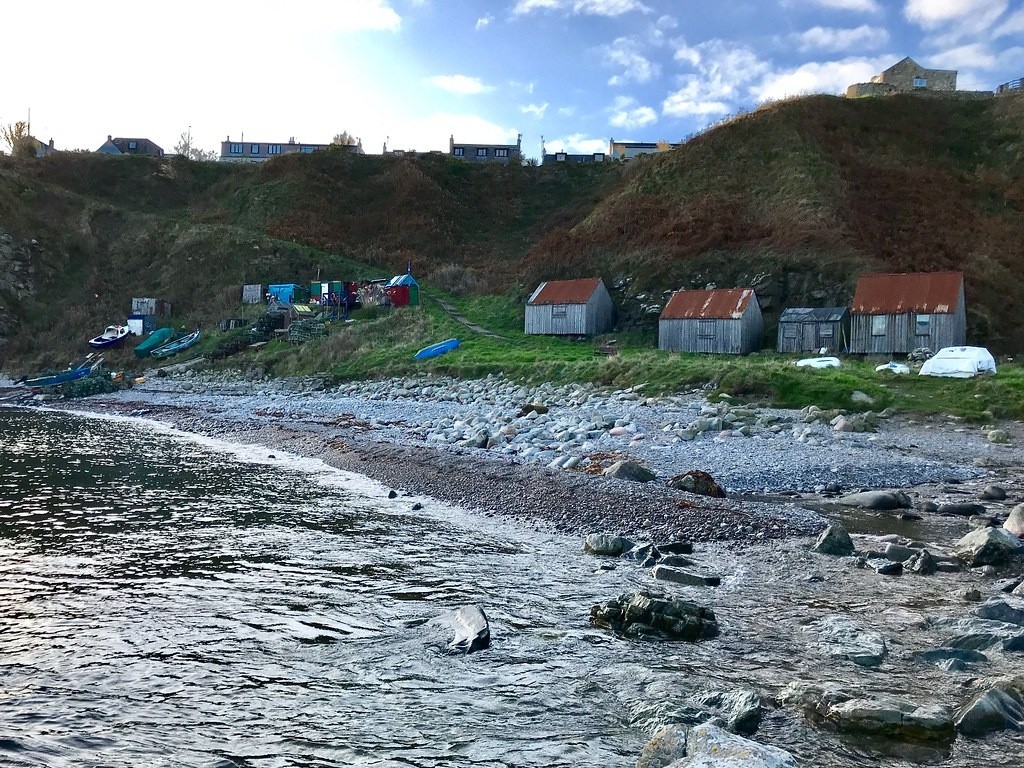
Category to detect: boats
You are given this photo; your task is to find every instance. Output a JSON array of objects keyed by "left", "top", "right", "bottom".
[
  {"left": 155, "top": 331, "right": 200, "bottom": 359},
  {"left": 137, "top": 327, "right": 173, "bottom": 357},
  {"left": 91, "top": 322, "right": 131, "bottom": 347},
  {"left": 412, "top": 338, "right": 461, "bottom": 361},
  {"left": 19, "top": 352, "right": 108, "bottom": 395}
]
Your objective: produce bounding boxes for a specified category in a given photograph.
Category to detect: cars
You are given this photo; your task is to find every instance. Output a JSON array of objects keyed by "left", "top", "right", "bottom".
[{"left": 918, "top": 346, "right": 995, "bottom": 380}]
